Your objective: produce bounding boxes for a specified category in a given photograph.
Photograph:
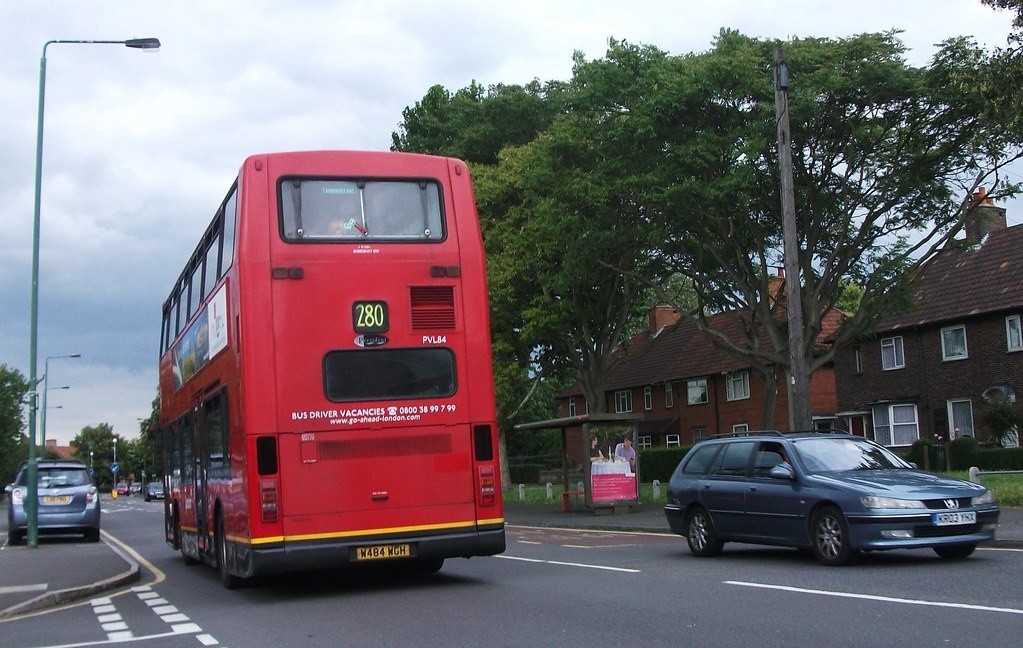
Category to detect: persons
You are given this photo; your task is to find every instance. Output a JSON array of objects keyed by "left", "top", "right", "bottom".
[
  {"left": 615, "top": 436, "right": 635, "bottom": 472},
  {"left": 125, "top": 480, "right": 130, "bottom": 496},
  {"left": 589, "top": 434, "right": 605, "bottom": 462}
]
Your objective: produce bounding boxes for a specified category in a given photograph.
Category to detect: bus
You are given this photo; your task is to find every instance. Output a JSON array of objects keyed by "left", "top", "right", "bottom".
[{"left": 148, "top": 150, "right": 506, "bottom": 590}]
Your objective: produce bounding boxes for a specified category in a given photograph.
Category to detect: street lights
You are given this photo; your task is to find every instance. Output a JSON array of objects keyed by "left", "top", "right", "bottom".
[
  {"left": 41, "top": 354, "right": 81, "bottom": 458},
  {"left": 26, "top": 37, "right": 161, "bottom": 543},
  {"left": 90, "top": 452, "right": 93, "bottom": 482},
  {"left": 113, "top": 439, "right": 117, "bottom": 488}
]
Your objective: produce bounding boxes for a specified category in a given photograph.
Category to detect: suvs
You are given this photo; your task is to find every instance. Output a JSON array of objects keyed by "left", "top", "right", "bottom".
[{"left": 664, "top": 430, "right": 999, "bottom": 565}]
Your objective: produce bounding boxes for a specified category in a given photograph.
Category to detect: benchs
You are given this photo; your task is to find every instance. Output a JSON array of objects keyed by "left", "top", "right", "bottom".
[{"left": 561, "top": 490, "right": 596, "bottom": 514}]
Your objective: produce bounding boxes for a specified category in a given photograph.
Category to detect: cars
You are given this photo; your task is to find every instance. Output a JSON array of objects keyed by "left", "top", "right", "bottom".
[
  {"left": 116, "top": 483, "right": 130, "bottom": 496},
  {"left": 130, "top": 482, "right": 142, "bottom": 493},
  {"left": 144, "top": 482, "right": 164, "bottom": 502},
  {"left": 5, "top": 459, "right": 100, "bottom": 545}
]
